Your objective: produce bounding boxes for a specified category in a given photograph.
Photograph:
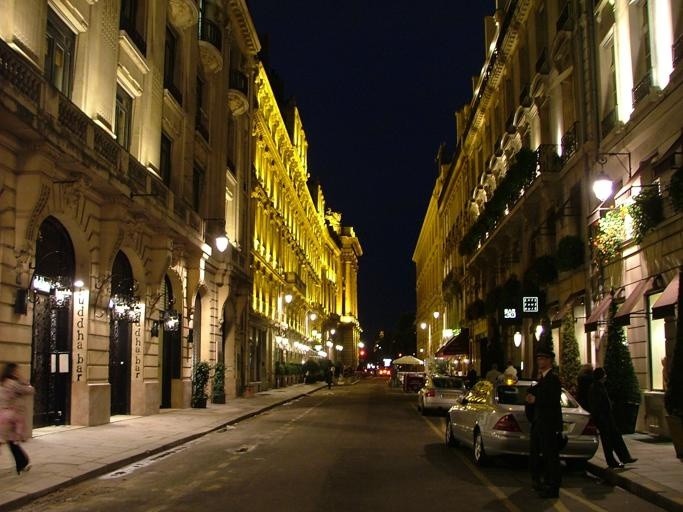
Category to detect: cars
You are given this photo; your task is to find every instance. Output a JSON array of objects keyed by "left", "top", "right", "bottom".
[
  {"left": 364, "top": 367, "right": 390, "bottom": 377},
  {"left": 442, "top": 379, "right": 598, "bottom": 467},
  {"left": 415, "top": 376, "right": 466, "bottom": 415}
]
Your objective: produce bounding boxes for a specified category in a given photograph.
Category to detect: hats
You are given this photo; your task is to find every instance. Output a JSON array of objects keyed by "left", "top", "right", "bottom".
[
  {"left": 592, "top": 367, "right": 606, "bottom": 381},
  {"left": 534, "top": 347, "right": 556, "bottom": 359}
]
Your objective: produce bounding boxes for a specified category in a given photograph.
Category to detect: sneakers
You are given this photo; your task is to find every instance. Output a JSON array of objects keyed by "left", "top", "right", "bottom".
[
  {"left": 622, "top": 458, "right": 639, "bottom": 464},
  {"left": 609, "top": 462, "right": 625, "bottom": 472}
]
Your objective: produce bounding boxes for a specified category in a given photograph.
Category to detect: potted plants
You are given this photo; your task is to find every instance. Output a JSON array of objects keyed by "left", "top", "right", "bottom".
[
  {"left": 192, "top": 362, "right": 209, "bottom": 408},
  {"left": 664, "top": 261, "right": 683, "bottom": 462},
  {"left": 603, "top": 303, "right": 640, "bottom": 433}
]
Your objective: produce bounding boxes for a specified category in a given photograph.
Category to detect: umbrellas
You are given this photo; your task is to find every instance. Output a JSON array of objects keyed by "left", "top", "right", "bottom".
[{"left": 392, "top": 355, "right": 424, "bottom": 372}]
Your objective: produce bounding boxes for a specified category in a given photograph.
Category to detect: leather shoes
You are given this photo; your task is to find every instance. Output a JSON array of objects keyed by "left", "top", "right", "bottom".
[{"left": 528, "top": 485, "right": 561, "bottom": 499}]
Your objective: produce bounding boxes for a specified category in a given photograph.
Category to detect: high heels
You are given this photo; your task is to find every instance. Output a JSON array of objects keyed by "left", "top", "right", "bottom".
[{"left": 17, "top": 463, "right": 33, "bottom": 477}]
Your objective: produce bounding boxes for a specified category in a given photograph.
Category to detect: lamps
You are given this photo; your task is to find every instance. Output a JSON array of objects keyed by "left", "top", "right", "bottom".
[
  {"left": 212, "top": 361, "right": 226, "bottom": 404},
  {"left": 26, "top": 251, "right": 72, "bottom": 315},
  {"left": 149, "top": 291, "right": 180, "bottom": 334},
  {"left": 590, "top": 149, "right": 630, "bottom": 203},
  {"left": 203, "top": 215, "right": 229, "bottom": 253},
  {"left": 92, "top": 274, "right": 141, "bottom": 324}
]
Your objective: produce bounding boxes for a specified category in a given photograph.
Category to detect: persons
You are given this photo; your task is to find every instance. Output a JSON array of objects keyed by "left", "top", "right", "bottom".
[
  {"left": 574, "top": 360, "right": 593, "bottom": 411},
  {"left": 325, "top": 366, "right": 332, "bottom": 388},
  {"left": 524, "top": 348, "right": 564, "bottom": 500},
  {"left": 588, "top": 366, "right": 638, "bottom": 468},
  {"left": 463, "top": 361, "right": 518, "bottom": 391},
  {"left": 0, "top": 362, "right": 35, "bottom": 473}
]
